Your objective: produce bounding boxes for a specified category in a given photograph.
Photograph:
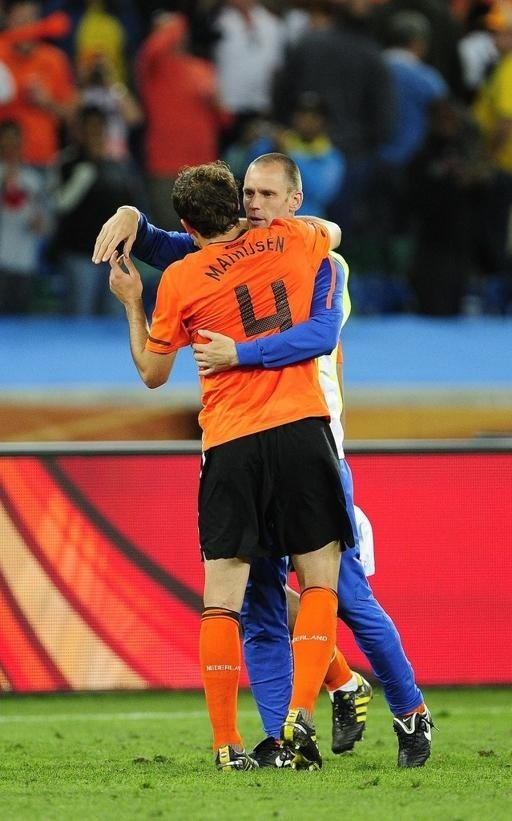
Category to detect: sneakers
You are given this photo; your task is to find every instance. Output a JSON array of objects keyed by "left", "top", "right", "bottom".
[
  {"left": 214, "top": 708, "right": 322, "bottom": 771},
  {"left": 327, "top": 670, "right": 373, "bottom": 755},
  {"left": 393, "top": 700, "right": 440, "bottom": 768}
]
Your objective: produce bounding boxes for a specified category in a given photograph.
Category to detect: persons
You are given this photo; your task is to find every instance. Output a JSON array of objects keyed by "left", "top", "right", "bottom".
[
  {"left": 0, "top": 0, "right": 512, "bottom": 323},
  {"left": 91, "top": 150, "right": 436, "bottom": 773},
  {"left": 107, "top": 159, "right": 359, "bottom": 777},
  {"left": 284, "top": 584, "right": 374, "bottom": 756}
]
